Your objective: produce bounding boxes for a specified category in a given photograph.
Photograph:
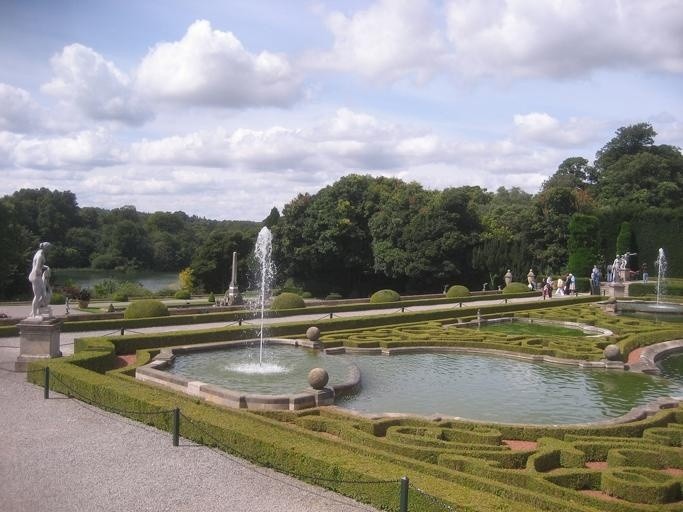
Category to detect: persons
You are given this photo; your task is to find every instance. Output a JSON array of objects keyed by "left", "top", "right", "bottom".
[
  {"left": 613, "top": 251, "right": 637, "bottom": 283},
  {"left": 642, "top": 259, "right": 648, "bottom": 283},
  {"left": 527, "top": 269, "right": 535, "bottom": 284},
  {"left": 29, "top": 242, "right": 52, "bottom": 319},
  {"left": 504, "top": 270, "right": 512, "bottom": 286},
  {"left": 543, "top": 262, "right": 613, "bottom": 298}
]
